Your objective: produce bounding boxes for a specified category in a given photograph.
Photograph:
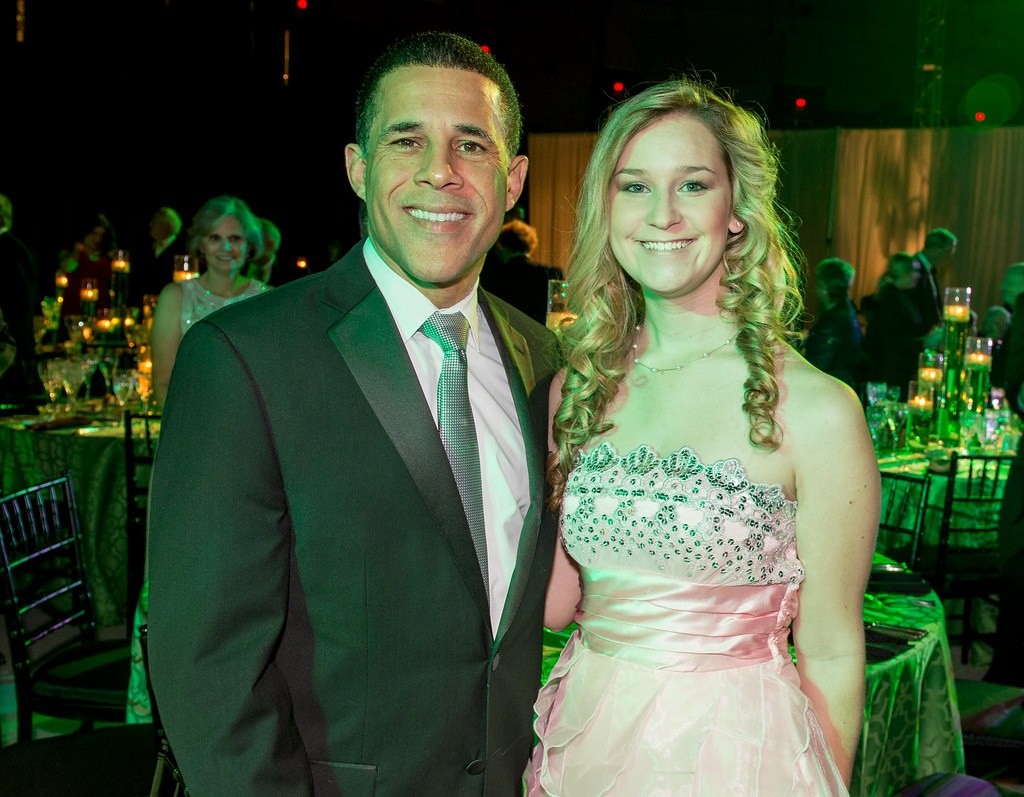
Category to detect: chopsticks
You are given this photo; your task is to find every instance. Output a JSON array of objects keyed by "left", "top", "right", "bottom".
[{"left": 863, "top": 620, "right": 928, "bottom": 641}]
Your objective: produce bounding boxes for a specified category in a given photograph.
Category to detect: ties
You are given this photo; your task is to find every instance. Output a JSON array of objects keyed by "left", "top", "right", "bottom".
[{"left": 419, "top": 313, "right": 492, "bottom": 607}]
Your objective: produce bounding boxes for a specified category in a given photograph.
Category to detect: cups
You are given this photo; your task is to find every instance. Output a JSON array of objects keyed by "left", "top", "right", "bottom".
[
  {"left": 174, "top": 254, "right": 198, "bottom": 281},
  {"left": 866, "top": 287, "right": 1015, "bottom": 462}
]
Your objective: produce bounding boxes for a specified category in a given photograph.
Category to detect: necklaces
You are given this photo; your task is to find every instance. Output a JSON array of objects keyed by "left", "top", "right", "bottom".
[{"left": 632, "top": 317, "right": 744, "bottom": 376}]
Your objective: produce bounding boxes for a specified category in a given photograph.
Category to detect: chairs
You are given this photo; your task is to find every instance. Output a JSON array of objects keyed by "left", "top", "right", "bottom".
[
  {"left": 124, "top": 407, "right": 163, "bottom": 636},
  {"left": 0, "top": 467, "right": 134, "bottom": 743},
  {"left": 877, "top": 469, "right": 933, "bottom": 572},
  {"left": 918, "top": 449, "right": 1018, "bottom": 668}
]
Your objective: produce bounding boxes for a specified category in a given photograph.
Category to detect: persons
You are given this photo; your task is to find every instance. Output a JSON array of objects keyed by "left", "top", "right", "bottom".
[
  {"left": 124, "top": 196, "right": 267, "bottom": 726},
  {"left": 521, "top": 74, "right": 884, "bottom": 797},
  {"left": 803, "top": 256, "right": 865, "bottom": 392},
  {"left": 0, "top": 185, "right": 279, "bottom": 382},
  {"left": 147, "top": 29, "right": 562, "bottom": 797},
  {"left": 982, "top": 292, "right": 1024, "bottom": 690},
  {"left": 487, "top": 216, "right": 567, "bottom": 325},
  {"left": 870, "top": 226, "right": 1024, "bottom": 420}
]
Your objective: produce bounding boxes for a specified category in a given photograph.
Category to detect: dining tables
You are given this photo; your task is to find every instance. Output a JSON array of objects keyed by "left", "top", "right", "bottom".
[
  {"left": 1, "top": 396, "right": 167, "bottom": 624},
  {"left": 124, "top": 551, "right": 966, "bottom": 797},
  {"left": 863, "top": 400, "right": 1024, "bottom": 553}
]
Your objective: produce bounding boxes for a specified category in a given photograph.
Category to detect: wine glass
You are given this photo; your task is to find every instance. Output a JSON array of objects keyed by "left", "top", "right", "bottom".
[
  {"left": 113, "top": 377, "right": 133, "bottom": 434},
  {"left": 136, "top": 373, "right": 152, "bottom": 414},
  {"left": 70, "top": 356, "right": 98, "bottom": 400},
  {"left": 60, "top": 364, "right": 84, "bottom": 411},
  {"left": 96, "top": 354, "right": 118, "bottom": 395},
  {"left": 37, "top": 362, "right": 68, "bottom": 409}
]
